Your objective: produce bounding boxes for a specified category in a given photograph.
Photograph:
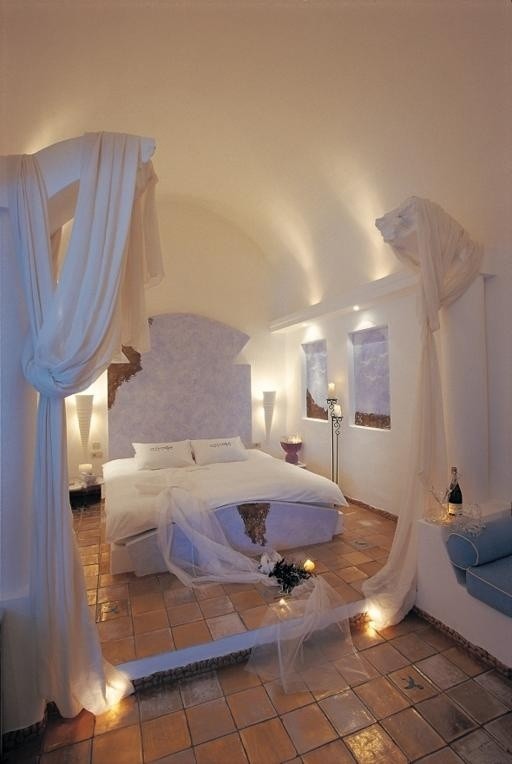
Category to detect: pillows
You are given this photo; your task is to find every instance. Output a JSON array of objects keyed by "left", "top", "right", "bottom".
[{"left": 130, "top": 434, "right": 251, "bottom": 472}]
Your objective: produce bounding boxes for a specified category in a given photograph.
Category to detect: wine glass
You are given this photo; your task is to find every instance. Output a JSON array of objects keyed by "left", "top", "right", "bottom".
[{"left": 431, "top": 488, "right": 449, "bottom": 517}]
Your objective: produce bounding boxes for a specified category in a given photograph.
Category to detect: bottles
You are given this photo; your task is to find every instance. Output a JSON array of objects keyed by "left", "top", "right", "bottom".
[{"left": 446, "top": 465, "right": 463, "bottom": 516}]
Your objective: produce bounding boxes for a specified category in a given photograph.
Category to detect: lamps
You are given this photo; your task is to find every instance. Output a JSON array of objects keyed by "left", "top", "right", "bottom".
[{"left": 327, "top": 382, "right": 343, "bottom": 482}]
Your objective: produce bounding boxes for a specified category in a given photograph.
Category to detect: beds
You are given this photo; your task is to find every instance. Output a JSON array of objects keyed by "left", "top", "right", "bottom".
[{"left": 103, "top": 449, "right": 344, "bottom": 577}]
